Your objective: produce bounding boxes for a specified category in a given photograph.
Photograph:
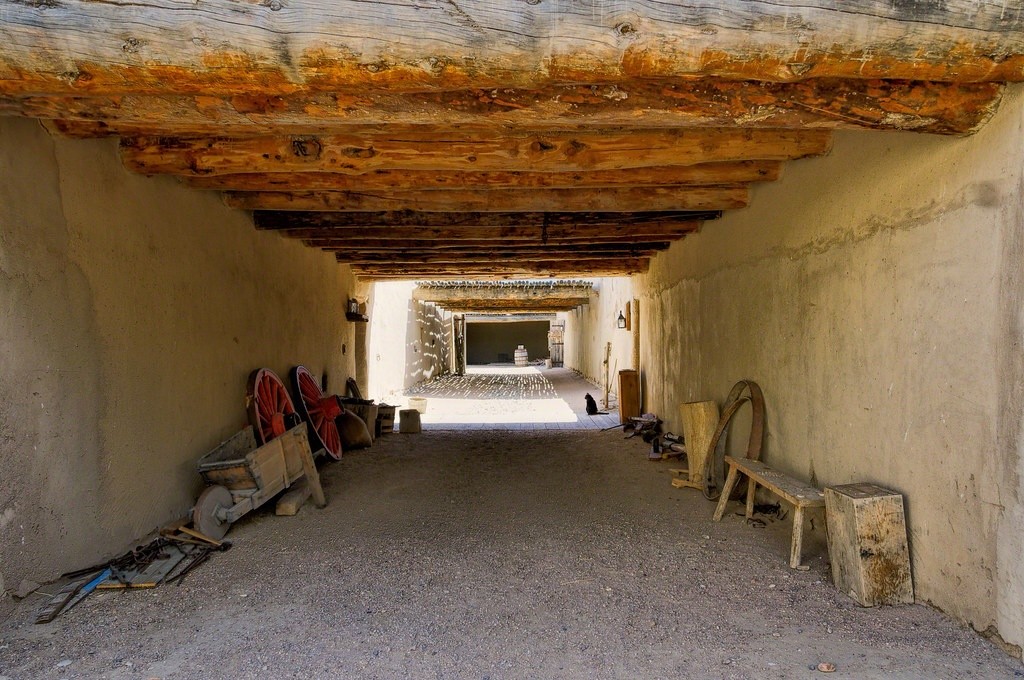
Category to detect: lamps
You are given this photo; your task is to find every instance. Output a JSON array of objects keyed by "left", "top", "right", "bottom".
[{"left": 618, "top": 311, "right": 626, "bottom": 328}]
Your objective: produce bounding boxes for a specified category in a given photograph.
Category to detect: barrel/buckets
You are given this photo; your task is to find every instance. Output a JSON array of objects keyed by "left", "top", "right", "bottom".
[
  {"left": 377, "top": 407, "right": 395, "bottom": 433},
  {"left": 514, "top": 349, "right": 528, "bottom": 367},
  {"left": 408, "top": 398, "right": 427, "bottom": 414}
]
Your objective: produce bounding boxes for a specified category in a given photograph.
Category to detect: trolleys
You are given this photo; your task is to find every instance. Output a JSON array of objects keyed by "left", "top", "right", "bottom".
[{"left": 190, "top": 422, "right": 326, "bottom": 543}]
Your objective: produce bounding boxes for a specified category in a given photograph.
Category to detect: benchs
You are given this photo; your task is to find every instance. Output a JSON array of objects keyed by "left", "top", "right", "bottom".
[{"left": 713, "top": 455, "right": 825, "bottom": 569}]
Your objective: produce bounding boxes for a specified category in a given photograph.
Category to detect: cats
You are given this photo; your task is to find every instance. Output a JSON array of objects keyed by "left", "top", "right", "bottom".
[{"left": 584, "top": 392, "right": 609, "bottom": 415}]
